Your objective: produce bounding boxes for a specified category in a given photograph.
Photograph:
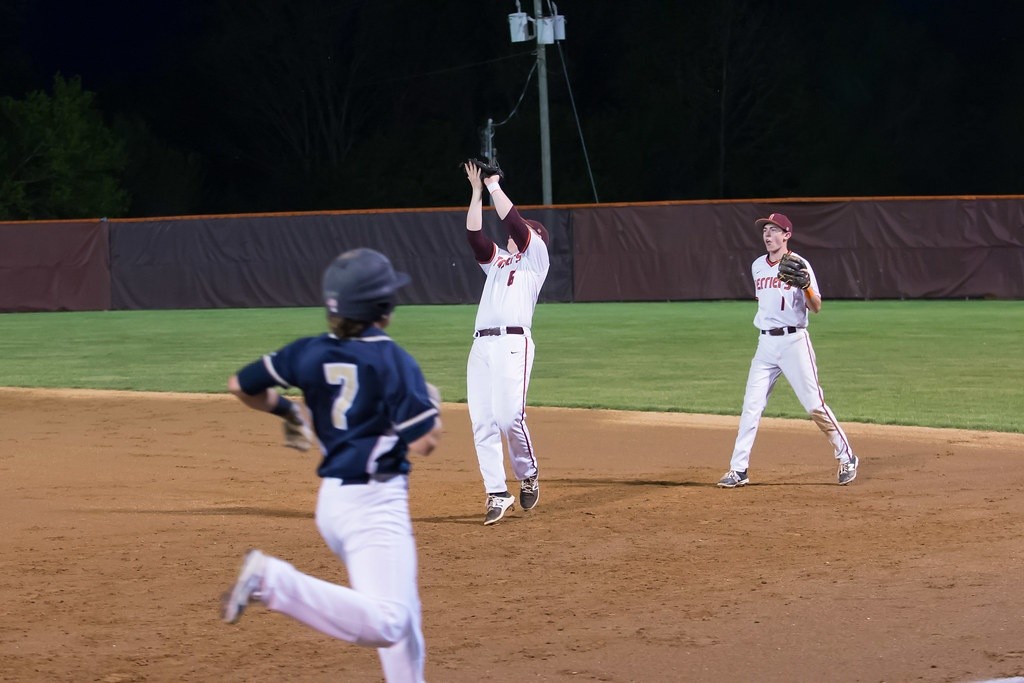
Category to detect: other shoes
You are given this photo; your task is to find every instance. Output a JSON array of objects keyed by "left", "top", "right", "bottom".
[{"left": 220, "top": 549, "right": 267, "bottom": 624}]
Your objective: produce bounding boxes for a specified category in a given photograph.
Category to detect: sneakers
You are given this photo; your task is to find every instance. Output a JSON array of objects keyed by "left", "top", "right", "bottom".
[
  {"left": 837, "top": 455, "right": 859, "bottom": 485},
  {"left": 717, "top": 468, "right": 749, "bottom": 488},
  {"left": 519, "top": 465, "right": 539, "bottom": 510},
  {"left": 483, "top": 491, "right": 515, "bottom": 525}
]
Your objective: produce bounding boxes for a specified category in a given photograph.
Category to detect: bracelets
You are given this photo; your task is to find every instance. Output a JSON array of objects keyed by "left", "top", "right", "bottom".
[
  {"left": 487, "top": 182, "right": 501, "bottom": 194},
  {"left": 269, "top": 396, "right": 292, "bottom": 418}
]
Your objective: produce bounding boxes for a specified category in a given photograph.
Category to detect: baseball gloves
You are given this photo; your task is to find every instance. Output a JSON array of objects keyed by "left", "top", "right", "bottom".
[
  {"left": 777, "top": 253, "right": 811, "bottom": 290},
  {"left": 459, "top": 158, "right": 504, "bottom": 182}
]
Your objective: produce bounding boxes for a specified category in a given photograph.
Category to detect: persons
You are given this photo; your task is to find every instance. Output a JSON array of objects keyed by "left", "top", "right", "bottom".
[
  {"left": 717, "top": 214, "right": 859, "bottom": 489},
  {"left": 465, "top": 160, "right": 550, "bottom": 527},
  {"left": 226, "top": 247, "right": 441, "bottom": 683}
]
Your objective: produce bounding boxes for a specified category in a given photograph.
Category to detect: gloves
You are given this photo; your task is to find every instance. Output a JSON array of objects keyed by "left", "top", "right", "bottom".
[
  {"left": 281, "top": 403, "right": 312, "bottom": 452},
  {"left": 427, "top": 382, "right": 442, "bottom": 409}
]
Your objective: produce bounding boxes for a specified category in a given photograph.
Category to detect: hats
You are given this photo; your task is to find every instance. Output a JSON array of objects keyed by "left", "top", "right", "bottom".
[
  {"left": 521, "top": 216, "right": 549, "bottom": 247},
  {"left": 321, "top": 248, "right": 411, "bottom": 319},
  {"left": 755, "top": 213, "right": 793, "bottom": 232}
]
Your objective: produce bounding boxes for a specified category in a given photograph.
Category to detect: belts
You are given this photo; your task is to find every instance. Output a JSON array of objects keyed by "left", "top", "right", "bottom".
[
  {"left": 762, "top": 327, "right": 796, "bottom": 335},
  {"left": 340, "top": 475, "right": 369, "bottom": 485},
  {"left": 473, "top": 327, "right": 523, "bottom": 338}
]
